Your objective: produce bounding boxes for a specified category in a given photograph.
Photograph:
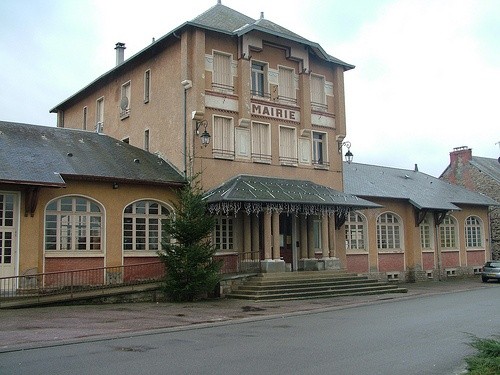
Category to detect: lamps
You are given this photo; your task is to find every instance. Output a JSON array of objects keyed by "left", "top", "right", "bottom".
[
  {"left": 337, "top": 141, "right": 354, "bottom": 165},
  {"left": 195, "top": 120, "right": 211, "bottom": 148}
]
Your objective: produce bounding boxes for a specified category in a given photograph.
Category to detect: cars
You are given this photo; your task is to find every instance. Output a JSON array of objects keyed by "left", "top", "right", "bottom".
[{"left": 481, "top": 261, "right": 500, "bottom": 283}]
